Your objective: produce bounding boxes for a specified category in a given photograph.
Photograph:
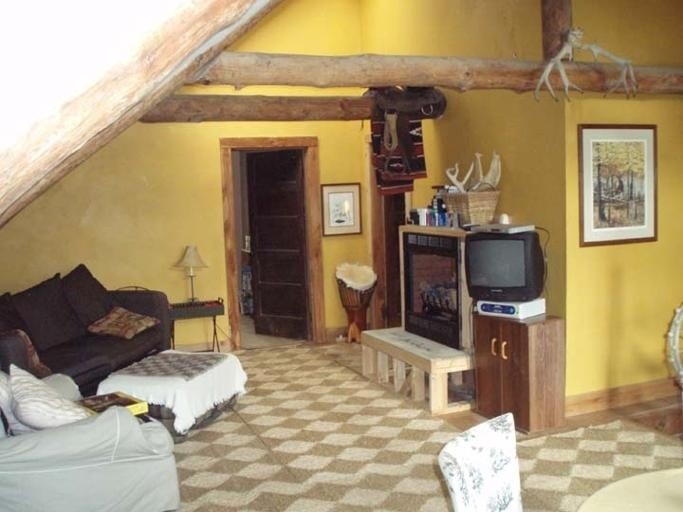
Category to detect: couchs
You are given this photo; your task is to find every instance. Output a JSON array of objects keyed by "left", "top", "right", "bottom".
[{"left": 1, "top": 291, "right": 175, "bottom": 395}]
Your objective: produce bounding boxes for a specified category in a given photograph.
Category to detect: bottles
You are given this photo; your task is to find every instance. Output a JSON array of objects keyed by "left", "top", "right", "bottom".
[{"left": 426, "top": 193, "right": 455, "bottom": 227}]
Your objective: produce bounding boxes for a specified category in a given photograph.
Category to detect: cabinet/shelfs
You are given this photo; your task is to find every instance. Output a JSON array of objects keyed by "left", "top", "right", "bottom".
[{"left": 474, "top": 316, "right": 566, "bottom": 432}]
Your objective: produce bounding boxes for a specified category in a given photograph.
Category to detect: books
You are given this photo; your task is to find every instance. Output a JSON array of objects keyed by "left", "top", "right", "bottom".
[{"left": 72, "top": 388, "right": 149, "bottom": 421}]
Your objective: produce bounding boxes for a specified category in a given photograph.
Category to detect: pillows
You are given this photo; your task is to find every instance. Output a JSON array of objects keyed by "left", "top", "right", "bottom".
[
  {"left": 10, "top": 364, "right": 90, "bottom": 430},
  {"left": 13, "top": 263, "right": 158, "bottom": 375}
]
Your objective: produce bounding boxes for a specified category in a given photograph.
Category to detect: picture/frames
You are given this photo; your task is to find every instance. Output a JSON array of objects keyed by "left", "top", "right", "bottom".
[
  {"left": 577, "top": 124, "right": 658, "bottom": 248},
  {"left": 320, "top": 183, "right": 362, "bottom": 236}
]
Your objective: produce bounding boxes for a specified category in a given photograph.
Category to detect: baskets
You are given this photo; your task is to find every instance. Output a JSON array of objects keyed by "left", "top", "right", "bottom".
[{"left": 444, "top": 182, "right": 499, "bottom": 225}]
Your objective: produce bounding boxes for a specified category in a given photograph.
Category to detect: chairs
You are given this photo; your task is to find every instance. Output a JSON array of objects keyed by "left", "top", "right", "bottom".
[
  {"left": 0, "top": 372, "right": 180, "bottom": 511},
  {"left": 437, "top": 411, "right": 523, "bottom": 511}
]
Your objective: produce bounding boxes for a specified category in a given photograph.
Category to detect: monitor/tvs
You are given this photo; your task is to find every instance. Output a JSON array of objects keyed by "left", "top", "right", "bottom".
[{"left": 465, "top": 230, "right": 544, "bottom": 301}]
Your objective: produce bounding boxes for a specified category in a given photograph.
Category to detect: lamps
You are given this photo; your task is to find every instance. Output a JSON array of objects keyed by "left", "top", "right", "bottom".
[{"left": 174, "top": 245, "right": 209, "bottom": 300}]
